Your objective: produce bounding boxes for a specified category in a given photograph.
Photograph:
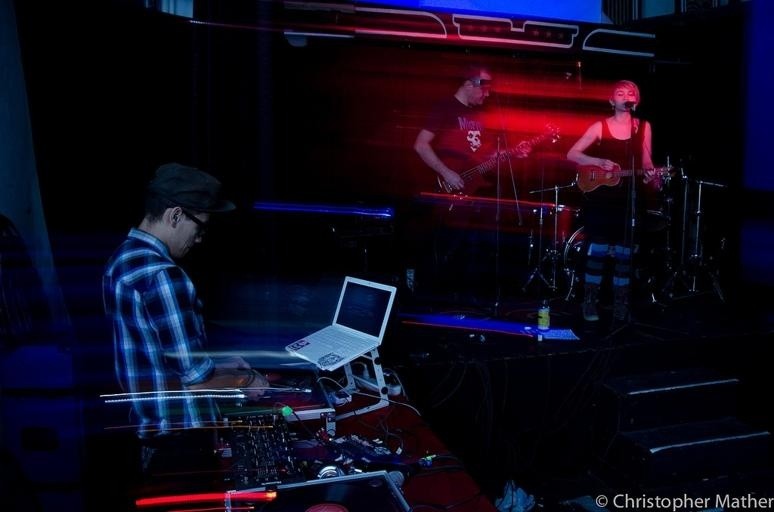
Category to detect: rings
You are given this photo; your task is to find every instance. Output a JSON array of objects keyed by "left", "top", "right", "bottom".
[
  {"left": 603, "top": 165, "right": 607, "bottom": 168},
  {"left": 454, "top": 184, "right": 458, "bottom": 188}
]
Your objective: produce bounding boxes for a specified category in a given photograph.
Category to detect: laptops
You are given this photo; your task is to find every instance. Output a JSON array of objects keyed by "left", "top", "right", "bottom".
[{"left": 285, "top": 274, "right": 399, "bottom": 373}]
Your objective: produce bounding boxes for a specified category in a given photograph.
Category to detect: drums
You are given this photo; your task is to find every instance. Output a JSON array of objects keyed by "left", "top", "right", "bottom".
[
  {"left": 532, "top": 208, "right": 580, "bottom": 262},
  {"left": 564, "top": 225, "right": 585, "bottom": 282}
]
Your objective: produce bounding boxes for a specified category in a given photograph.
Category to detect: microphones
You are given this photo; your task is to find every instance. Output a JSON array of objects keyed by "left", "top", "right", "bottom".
[{"left": 624, "top": 101, "right": 635, "bottom": 110}]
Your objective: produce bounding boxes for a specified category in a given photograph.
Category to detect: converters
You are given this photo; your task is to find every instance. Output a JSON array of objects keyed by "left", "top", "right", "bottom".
[{"left": 329, "top": 390, "right": 353, "bottom": 405}]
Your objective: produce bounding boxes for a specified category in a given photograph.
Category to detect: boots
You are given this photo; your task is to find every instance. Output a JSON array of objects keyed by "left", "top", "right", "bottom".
[
  {"left": 581, "top": 282, "right": 601, "bottom": 321},
  {"left": 612, "top": 284, "right": 634, "bottom": 322}
]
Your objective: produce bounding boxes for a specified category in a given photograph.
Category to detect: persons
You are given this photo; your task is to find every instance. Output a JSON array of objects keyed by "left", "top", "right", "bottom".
[
  {"left": 567, "top": 79, "right": 664, "bottom": 324},
  {"left": 413, "top": 65, "right": 528, "bottom": 344},
  {"left": 102, "top": 164, "right": 268, "bottom": 474}
]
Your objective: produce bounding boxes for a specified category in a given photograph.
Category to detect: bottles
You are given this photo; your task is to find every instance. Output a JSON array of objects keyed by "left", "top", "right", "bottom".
[{"left": 537, "top": 298, "right": 551, "bottom": 332}]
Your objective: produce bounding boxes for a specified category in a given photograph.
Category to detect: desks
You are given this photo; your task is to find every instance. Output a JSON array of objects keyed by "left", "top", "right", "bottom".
[{"left": 212, "top": 361, "right": 497, "bottom": 511}]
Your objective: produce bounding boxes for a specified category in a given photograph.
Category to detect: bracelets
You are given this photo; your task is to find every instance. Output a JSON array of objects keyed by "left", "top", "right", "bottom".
[{"left": 239, "top": 370, "right": 255, "bottom": 388}]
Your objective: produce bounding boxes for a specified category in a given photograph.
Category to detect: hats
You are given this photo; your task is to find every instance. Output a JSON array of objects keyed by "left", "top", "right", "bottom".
[{"left": 149, "top": 162, "right": 239, "bottom": 215}]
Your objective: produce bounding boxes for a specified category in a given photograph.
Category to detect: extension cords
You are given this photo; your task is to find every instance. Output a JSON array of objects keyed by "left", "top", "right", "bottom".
[{"left": 355, "top": 374, "right": 401, "bottom": 396}]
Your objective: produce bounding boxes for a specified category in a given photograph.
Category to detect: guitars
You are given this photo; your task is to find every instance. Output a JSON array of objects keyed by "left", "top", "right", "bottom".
[
  {"left": 434, "top": 120, "right": 562, "bottom": 207},
  {"left": 576, "top": 165, "right": 676, "bottom": 192}
]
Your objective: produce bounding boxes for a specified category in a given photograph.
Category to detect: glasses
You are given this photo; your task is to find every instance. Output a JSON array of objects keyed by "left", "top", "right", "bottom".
[{"left": 165, "top": 204, "right": 213, "bottom": 239}]
